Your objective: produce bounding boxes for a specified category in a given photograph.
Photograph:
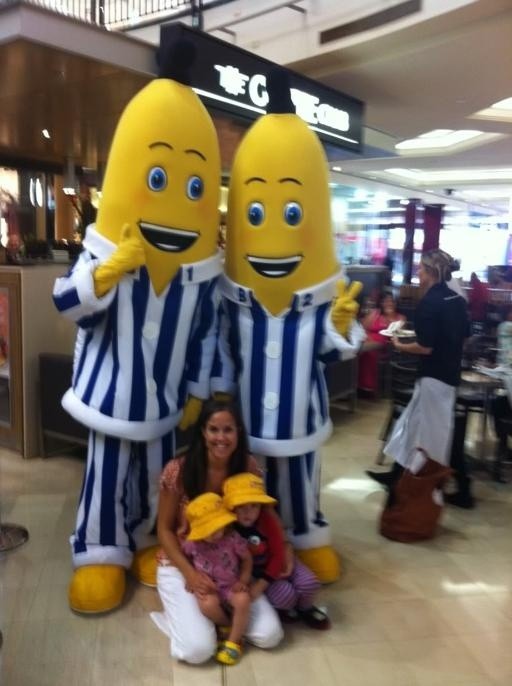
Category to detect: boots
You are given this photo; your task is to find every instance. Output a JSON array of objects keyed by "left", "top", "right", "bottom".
[
  {"left": 444, "top": 476, "right": 473, "bottom": 509},
  {"left": 365, "top": 459, "right": 403, "bottom": 494}
]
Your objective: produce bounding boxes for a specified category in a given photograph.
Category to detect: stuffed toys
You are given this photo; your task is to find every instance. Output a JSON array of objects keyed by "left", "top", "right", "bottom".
[{"left": 52, "top": 35, "right": 367, "bottom": 614}]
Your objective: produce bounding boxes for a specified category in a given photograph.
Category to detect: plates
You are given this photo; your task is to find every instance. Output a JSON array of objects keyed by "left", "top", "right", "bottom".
[{"left": 379, "top": 328, "right": 415, "bottom": 338}]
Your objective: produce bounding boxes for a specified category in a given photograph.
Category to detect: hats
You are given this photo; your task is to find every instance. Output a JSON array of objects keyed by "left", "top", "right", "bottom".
[
  {"left": 185, "top": 493, "right": 235, "bottom": 539},
  {"left": 224, "top": 475, "right": 276, "bottom": 508}
]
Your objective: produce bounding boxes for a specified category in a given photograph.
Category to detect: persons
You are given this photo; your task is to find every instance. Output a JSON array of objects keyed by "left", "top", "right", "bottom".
[
  {"left": 151, "top": 397, "right": 330, "bottom": 666},
  {"left": 359, "top": 249, "right": 491, "bottom": 509}
]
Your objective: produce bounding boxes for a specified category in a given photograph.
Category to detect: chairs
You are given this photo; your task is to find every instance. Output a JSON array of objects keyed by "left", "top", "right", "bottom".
[{"left": 376, "top": 360, "right": 468, "bottom": 477}]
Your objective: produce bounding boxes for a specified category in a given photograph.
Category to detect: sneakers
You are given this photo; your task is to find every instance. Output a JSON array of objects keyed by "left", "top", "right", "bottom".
[
  {"left": 300, "top": 546, "right": 342, "bottom": 582},
  {"left": 71, "top": 565, "right": 123, "bottom": 616},
  {"left": 137, "top": 547, "right": 162, "bottom": 590},
  {"left": 218, "top": 642, "right": 240, "bottom": 662},
  {"left": 301, "top": 608, "right": 327, "bottom": 627},
  {"left": 219, "top": 625, "right": 229, "bottom": 633},
  {"left": 279, "top": 611, "right": 294, "bottom": 622}
]
